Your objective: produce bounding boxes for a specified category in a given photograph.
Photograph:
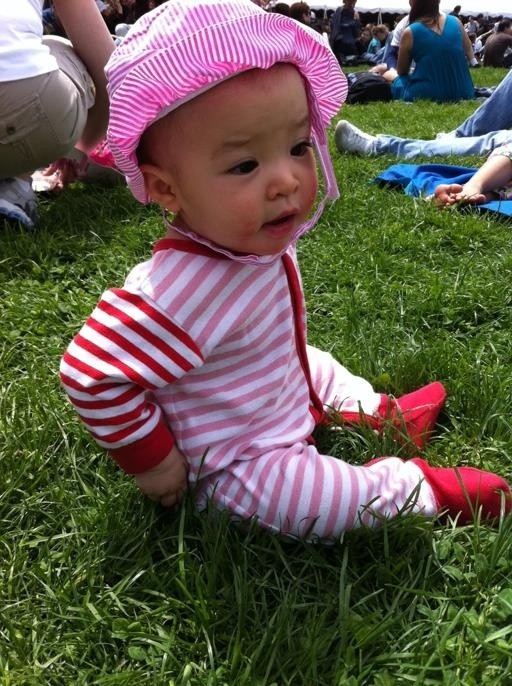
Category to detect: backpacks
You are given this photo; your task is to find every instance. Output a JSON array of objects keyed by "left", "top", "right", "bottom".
[{"left": 345, "top": 71, "right": 393, "bottom": 105}]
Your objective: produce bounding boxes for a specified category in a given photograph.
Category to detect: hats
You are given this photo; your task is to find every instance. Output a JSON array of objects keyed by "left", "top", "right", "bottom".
[{"left": 89, "top": 0, "right": 349, "bottom": 204}]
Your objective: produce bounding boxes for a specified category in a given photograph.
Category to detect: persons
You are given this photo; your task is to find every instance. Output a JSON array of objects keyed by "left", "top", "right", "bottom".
[
  {"left": 54, "top": 0, "right": 512, "bottom": 551},
  {"left": 0, "top": 0, "right": 166, "bottom": 231},
  {"left": 436, "top": 68, "right": 512, "bottom": 138},
  {"left": 334, "top": 120, "right": 512, "bottom": 208},
  {"left": 253, "top": 1, "right": 512, "bottom": 70},
  {"left": 368, "top": 1, "right": 479, "bottom": 102}
]
